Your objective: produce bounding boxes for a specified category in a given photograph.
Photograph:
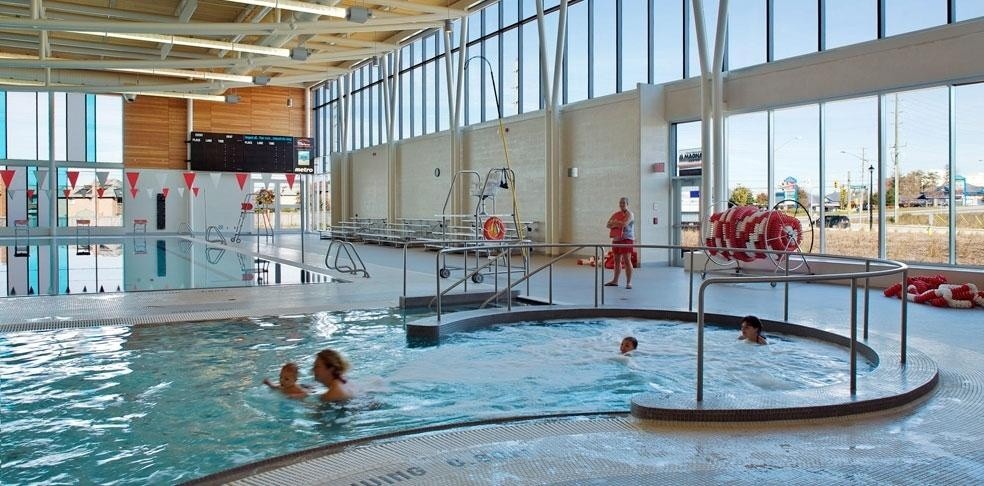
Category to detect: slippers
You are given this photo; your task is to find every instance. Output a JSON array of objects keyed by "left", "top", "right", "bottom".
[
  {"left": 626, "top": 285, "right": 632, "bottom": 289},
  {"left": 604, "top": 282, "right": 618, "bottom": 286}
]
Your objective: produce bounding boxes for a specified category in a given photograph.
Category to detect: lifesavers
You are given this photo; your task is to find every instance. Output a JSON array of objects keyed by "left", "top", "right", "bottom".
[{"left": 483, "top": 218, "right": 505, "bottom": 240}]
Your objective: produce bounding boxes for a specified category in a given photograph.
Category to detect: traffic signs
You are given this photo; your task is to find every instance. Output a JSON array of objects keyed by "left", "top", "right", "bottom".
[{"left": 849, "top": 185, "right": 866, "bottom": 189}]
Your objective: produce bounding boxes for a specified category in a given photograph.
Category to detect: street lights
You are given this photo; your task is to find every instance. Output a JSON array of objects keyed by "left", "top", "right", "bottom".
[
  {"left": 841, "top": 148, "right": 865, "bottom": 230},
  {"left": 869, "top": 165, "right": 875, "bottom": 230}
]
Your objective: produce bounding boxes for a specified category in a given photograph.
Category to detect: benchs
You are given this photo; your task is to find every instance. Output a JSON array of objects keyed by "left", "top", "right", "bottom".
[{"left": 319, "top": 218, "right": 537, "bottom": 256}]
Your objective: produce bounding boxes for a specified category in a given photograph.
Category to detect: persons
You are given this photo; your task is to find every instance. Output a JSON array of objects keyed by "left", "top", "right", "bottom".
[
  {"left": 619, "top": 337, "right": 639, "bottom": 353},
  {"left": 260, "top": 362, "right": 307, "bottom": 399},
  {"left": 737, "top": 315, "right": 769, "bottom": 344},
  {"left": 312, "top": 349, "right": 354, "bottom": 400},
  {"left": 604, "top": 197, "right": 635, "bottom": 289}
]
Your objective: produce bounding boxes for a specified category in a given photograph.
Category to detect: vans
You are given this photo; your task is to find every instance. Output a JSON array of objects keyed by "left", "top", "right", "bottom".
[{"left": 809, "top": 215, "right": 849, "bottom": 228}]
